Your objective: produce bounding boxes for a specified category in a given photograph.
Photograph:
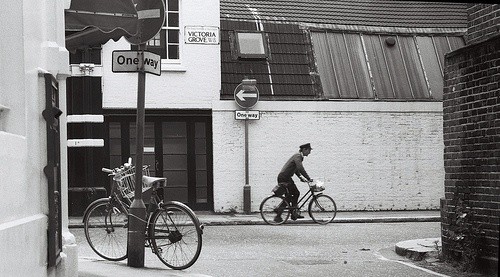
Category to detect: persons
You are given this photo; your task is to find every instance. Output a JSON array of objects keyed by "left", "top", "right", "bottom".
[{"left": 273, "top": 142, "right": 313, "bottom": 222}]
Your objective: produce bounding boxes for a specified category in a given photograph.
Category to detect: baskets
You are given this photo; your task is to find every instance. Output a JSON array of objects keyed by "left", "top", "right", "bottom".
[
  {"left": 309, "top": 183, "right": 325, "bottom": 192},
  {"left": 112, "top": 165, "right": 152, "bottom": 199}
]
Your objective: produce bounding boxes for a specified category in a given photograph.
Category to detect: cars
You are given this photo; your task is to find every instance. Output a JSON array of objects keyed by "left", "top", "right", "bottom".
[
  {"left": 259, "top": 178, "right": 338, "bottom": 226},
  {"left": 81, "top": 157, "right": 204, "bottom": 270}
]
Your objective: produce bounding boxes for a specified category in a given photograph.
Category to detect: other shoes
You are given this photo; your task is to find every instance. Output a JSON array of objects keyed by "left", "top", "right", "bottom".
[
  {"left": 275, "top": 216, "right": 283, "bottom": 221},
  {"left": 297, "top": 215, "right": 304, "bottom": 218}
]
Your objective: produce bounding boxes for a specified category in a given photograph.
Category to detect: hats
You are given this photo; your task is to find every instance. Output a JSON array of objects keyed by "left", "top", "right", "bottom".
[{"left": 300, "top": 143, "right": 313, "bottom": 150}]
